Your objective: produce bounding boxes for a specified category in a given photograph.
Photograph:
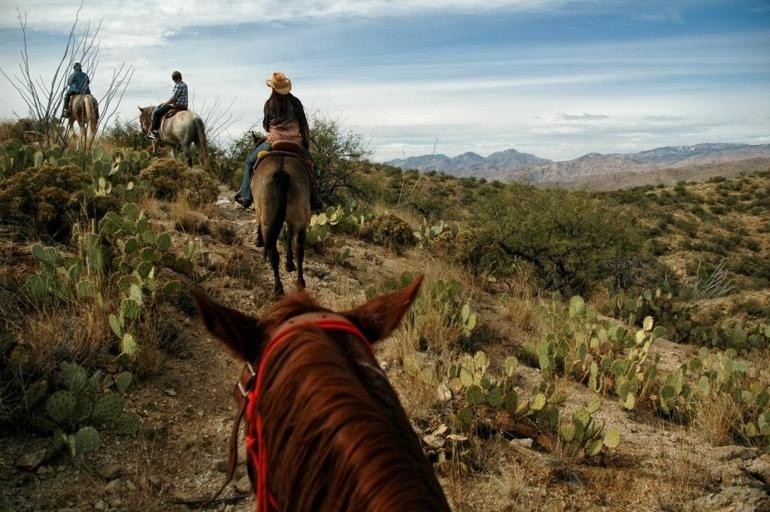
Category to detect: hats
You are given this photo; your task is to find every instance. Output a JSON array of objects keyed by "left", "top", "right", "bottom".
[{"left": 266, "top": 73, "right": 291, "bottom": 96}]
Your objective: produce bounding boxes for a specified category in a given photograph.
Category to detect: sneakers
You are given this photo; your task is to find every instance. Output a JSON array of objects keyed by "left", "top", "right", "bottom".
[
  {"left": 63, "top": 112, "right": 69, "bottom": 118},
  {"left": 148, "top": 132, "right": 157, "bottom": 139}
]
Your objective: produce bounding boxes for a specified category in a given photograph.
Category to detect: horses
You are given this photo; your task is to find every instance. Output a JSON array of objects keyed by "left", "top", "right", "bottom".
[
  {"left": 136, "top": 104, "right": 210, "bottom": 171},
  {"left": 244, "top": 128, "right": 317, "bottom": 299},
  {"left": 65, "top": 91, "right": 99, "bottom": 141},
  {"left": 187, "top": 270, "right": 453, "bottom": 512}
]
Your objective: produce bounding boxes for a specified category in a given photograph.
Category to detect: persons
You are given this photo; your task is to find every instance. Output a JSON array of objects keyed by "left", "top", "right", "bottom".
[
  {"left": 146, "top": 71, "right": 188, "bottom": 138},
  {"left": 62, "top": 62, "right": 90, "bottom": 117},
  {"left": 231, "top": 72, "right": 323, "bottom": 210}
]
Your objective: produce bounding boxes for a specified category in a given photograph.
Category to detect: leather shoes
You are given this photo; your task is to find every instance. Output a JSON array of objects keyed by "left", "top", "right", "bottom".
[{"left": 236, "top": 196, "right": 250, "bottom": 207}]
